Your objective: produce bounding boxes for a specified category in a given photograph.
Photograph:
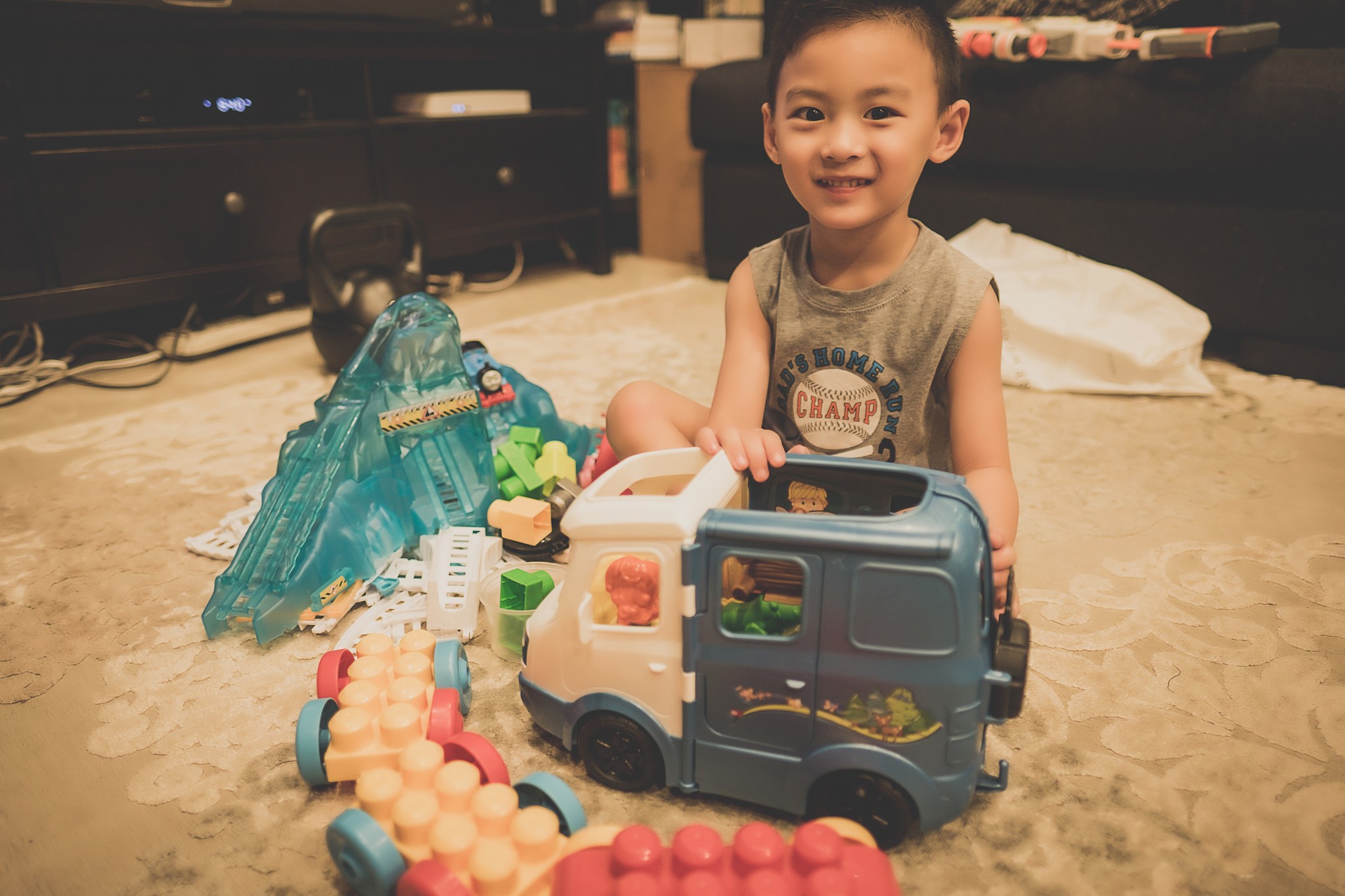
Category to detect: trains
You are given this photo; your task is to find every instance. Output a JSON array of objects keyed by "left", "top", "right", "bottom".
[{"left": 458, "top": 341, "right": 517, "bottom": 414}]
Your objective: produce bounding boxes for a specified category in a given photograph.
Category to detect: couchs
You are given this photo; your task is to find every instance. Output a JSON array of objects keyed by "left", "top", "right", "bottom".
[{"left": 688, "top": 0, "right": 1345, "bottom": 388}]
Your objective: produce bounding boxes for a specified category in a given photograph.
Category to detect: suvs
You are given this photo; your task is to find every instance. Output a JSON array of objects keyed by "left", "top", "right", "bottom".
[{"left": 516, "top": 448, "right": 1031, "bottom": 852}]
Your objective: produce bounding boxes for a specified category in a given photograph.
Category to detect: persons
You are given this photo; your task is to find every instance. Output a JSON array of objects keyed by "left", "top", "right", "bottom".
[{"left": 605, "top": 0, "right": 1023, "bottom": 620}]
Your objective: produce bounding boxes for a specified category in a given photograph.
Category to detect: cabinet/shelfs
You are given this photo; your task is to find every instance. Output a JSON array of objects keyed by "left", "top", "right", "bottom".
[{"left": 0, "top": 0, "right": 612, "bottom": 340}]
